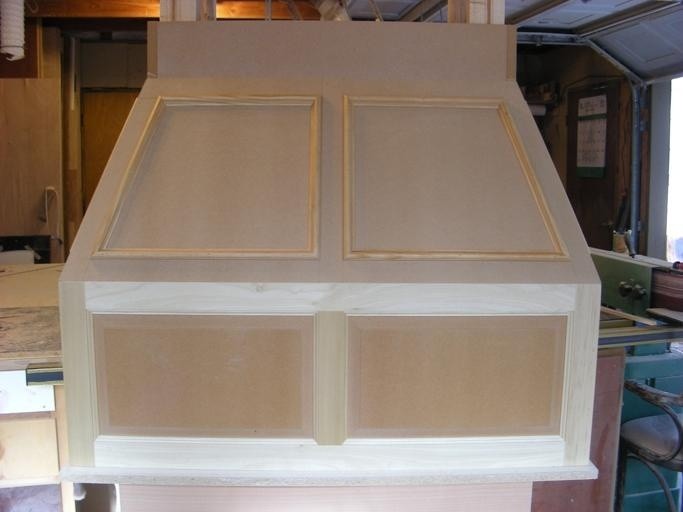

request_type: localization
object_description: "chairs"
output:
[616,380,683,512]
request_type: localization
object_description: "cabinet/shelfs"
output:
[618,350,683,511]
[532,346,625,510]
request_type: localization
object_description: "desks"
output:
[0,263,77,512]
